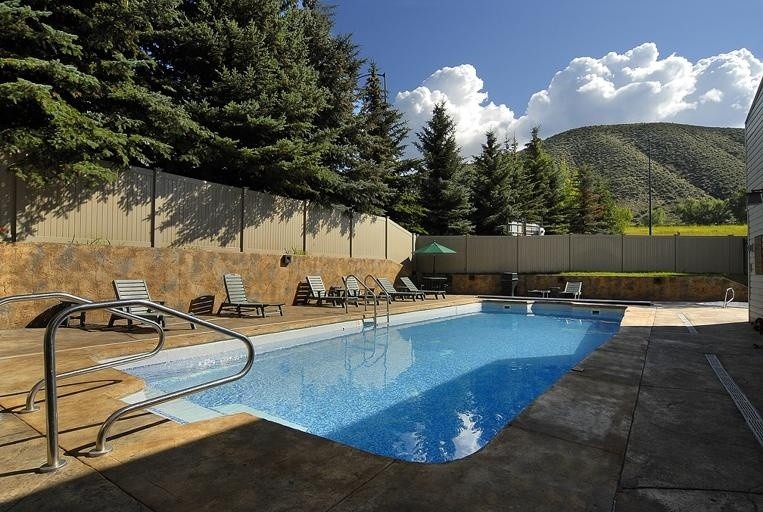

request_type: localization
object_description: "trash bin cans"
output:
[500,272,518,296]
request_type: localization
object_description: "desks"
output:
[421,276,447,291]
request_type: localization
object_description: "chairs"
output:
[341,276,392,304]
[399,276,446,299]
[216,273,285,318]
[107,279,198,333]
[304,275,358,307]
[376,278,424,302]
[526,286,560,298]
[558,281,583,298]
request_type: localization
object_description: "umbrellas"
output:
[411,239,458,277]
